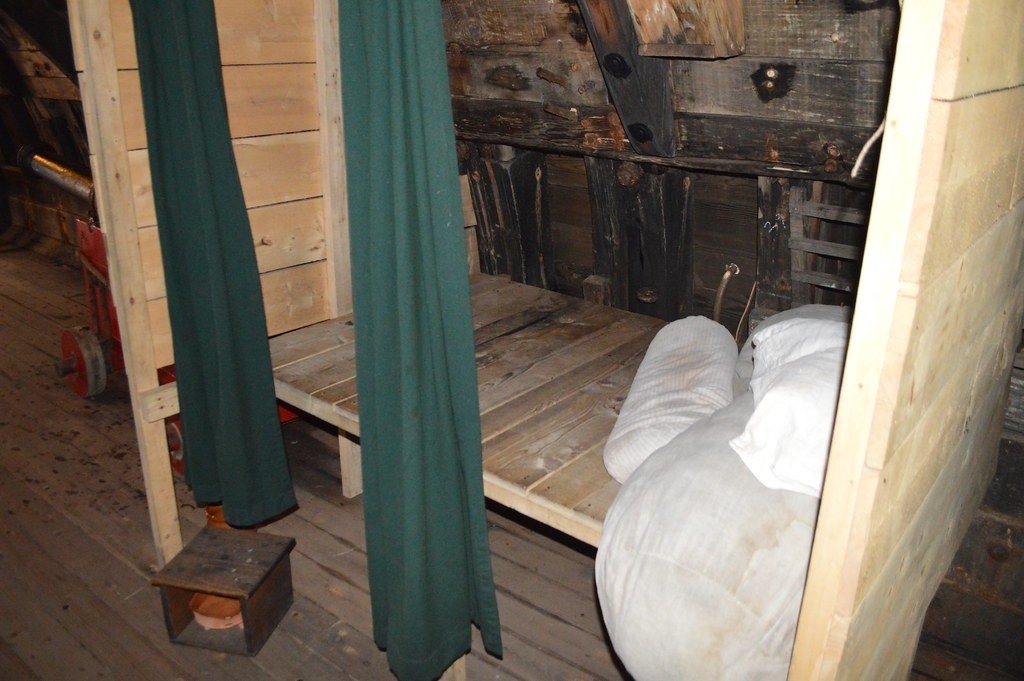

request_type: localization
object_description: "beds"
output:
[262,271,747,555]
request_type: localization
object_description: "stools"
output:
[147,525,297,658]
